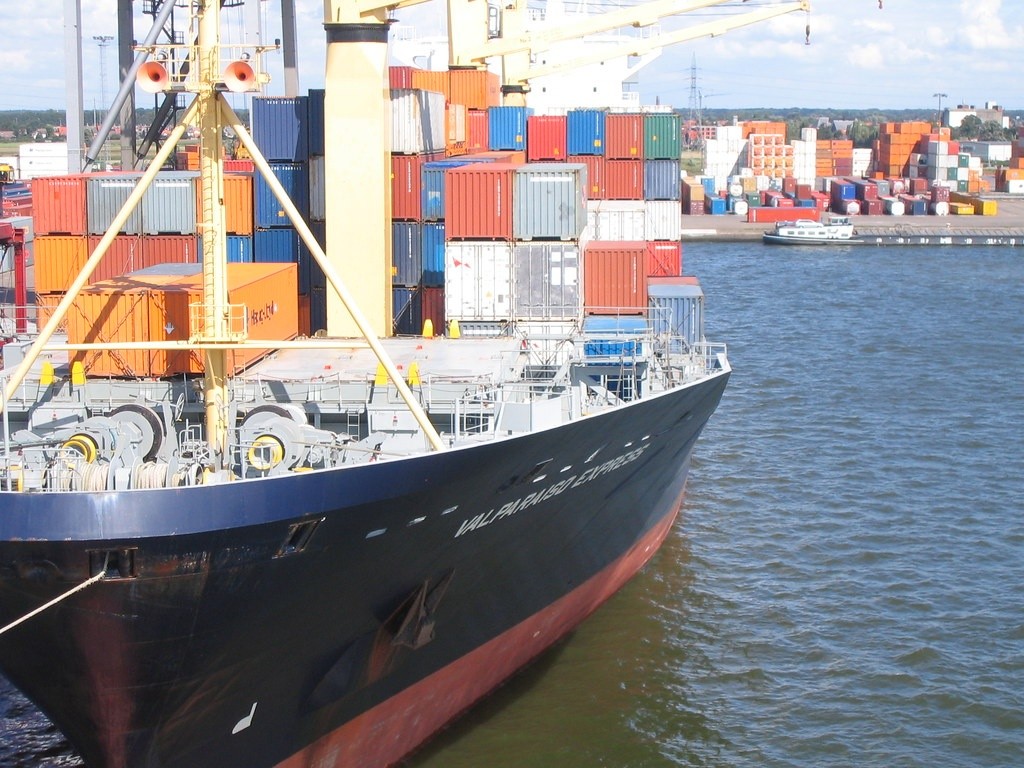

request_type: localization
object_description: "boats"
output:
[0,0,731,768]
[763,222,864,245]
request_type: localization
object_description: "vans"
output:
[829,217,851,226]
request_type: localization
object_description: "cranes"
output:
[325,0,883,340]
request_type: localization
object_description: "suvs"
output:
[796,219,824,228]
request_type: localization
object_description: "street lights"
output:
[933,92,948,187]
